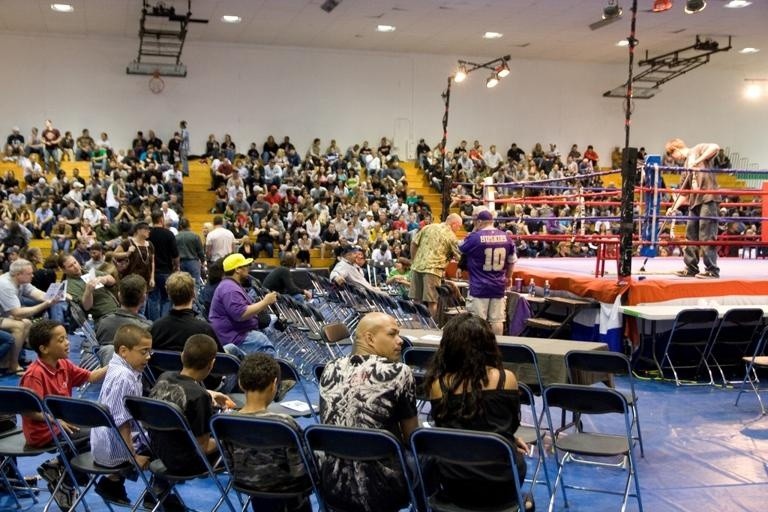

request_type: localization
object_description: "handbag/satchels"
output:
[111,257,129,273]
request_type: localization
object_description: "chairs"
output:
[735,326,768,415]
[657,309,718,387]
[699,307,764,388]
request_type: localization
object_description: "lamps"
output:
[453,53,511,88]
[685,0,706,14]
[602,0,623,20]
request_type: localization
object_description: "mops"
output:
[639,171,691,280]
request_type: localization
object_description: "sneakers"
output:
[0,475,37,490]
[675,270,695,277]
[144,491,189,512]
[37,459,76,512]
[274,379,296,402]
[695,271,719,279]
[19,357,32,365]
[95,476,131,504]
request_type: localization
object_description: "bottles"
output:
[544,280,550,298]
[529,278,535,297]
[217,400,231,413]
[455,268,464,281]
[87,312,95,330]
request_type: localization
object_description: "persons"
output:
[1,118,768,512]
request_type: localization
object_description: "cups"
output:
[516,278,523,293]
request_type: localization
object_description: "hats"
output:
[223,253,254,272]
[39,178,46,185]
[253,185,262,192]
[343,245,357,253]
[73,182,84,188]
[86,243,100,251]
[477,210,492,220]
[366,211,373,216]
[131,221,148,232]
[5,248,17,254]
